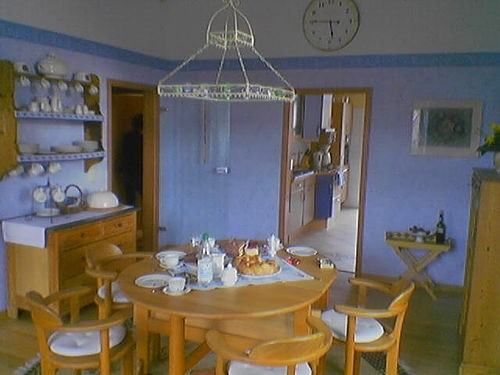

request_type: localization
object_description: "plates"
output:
[17,144,40,154]
[162,287,191,296]
[74,72,91,82]
[51,145,82,153]
[72,141,98,152]
[159,263,182,269]
[135,274,171,288]
[156,251,187,260]
[286,247,317,256]
[37,208,60,217]
[236,262,282,278]
[14,62,31,73]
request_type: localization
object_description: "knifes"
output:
[300,148,312,167]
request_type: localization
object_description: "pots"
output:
[34,51,74,79]
[35,96,49,108]
[341,95,350,103]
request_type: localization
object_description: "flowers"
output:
[475,123,500,157]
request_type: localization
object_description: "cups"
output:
[32,102,40,112]
[75,82,84,92]
[51,186,65,202]
[58,80,68,90]
[169,278,186,292]
[33,188,47,202]
[83,105,88,114]
[219,261,239,287]
[75,104,82,113]
[208,240,214,246]
[29,163,45,175]
[20,77,30,87]
[159,255,179,267]
[41,79,50,89]
[47,162,62,174]
[89,85,98,95]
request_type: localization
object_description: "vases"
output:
[494,152,500,175]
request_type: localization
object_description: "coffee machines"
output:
[323,147,333,170]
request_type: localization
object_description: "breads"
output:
[232,255,278,276]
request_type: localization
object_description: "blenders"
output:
[313,152,322,173]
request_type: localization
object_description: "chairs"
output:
[190,316,334,375]
[316,278,415,375]
[84,241,154,320]
[25,289,134,375]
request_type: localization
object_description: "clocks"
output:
[303,0,360,51]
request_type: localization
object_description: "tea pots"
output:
[56,184,82,213]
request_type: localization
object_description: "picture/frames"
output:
[410,99,482,157]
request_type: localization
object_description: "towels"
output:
[167,255,314,291]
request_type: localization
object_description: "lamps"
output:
[156,0,297,103]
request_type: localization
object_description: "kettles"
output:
[313,152,322,169]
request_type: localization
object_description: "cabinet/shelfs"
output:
[290,174,317,235]
[456,169,500,375]
[1,209,140,319]
[0,60,106,182]
[315,175,342,218]
[330,103,353,203]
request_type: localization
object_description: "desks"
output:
[384,232,452,300]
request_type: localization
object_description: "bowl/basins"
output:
[87,190,120,208]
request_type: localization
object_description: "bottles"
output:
[436,210,445,242]
[50,92,62,112]
[198,233,213,288]
[205,251,226,280]
[191,233,196,248]
[196,239,200,246]
[41,100,51,112]
